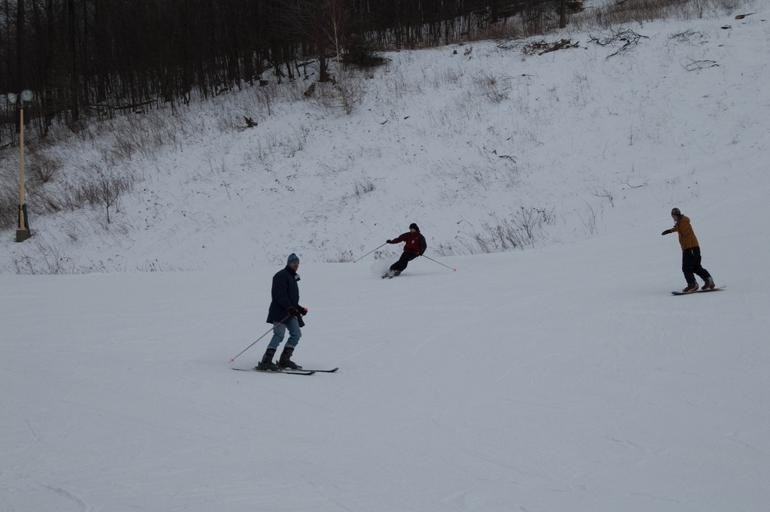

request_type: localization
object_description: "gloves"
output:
[416,251,421,256]
[662,230,671,235]
[387,240,392,243]
[286,305,307,316]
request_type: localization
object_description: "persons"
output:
[662,207,715,292]
[262,253,307,371]
[387,223,426,275]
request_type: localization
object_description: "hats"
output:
[671,208,680,213]
[410,223,418,228]
[287,253,299,261]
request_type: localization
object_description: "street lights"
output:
[6,85,34,242]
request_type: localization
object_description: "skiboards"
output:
[671,282,728,296]
[233,366,339,376]
[381,268,401,279]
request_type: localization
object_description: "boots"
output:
[382,269,400,279]
[257,346,303,371]
[702,275,715,290]
[683,277,699,292]
